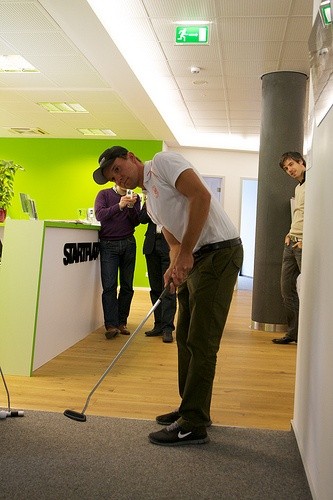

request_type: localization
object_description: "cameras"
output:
[127,189,132,199]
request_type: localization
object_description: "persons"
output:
[140,200,177,342]
[272,151,306,344]
[94,186,141,338]
[93,146,244,446]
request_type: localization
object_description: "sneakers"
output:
[272,333,297,344]
[155,409,213,427]
[148,416,210,446]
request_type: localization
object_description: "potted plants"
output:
[0,159,23,223]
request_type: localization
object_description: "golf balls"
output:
[0,410,6,419]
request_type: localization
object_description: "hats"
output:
[93,145,128,185]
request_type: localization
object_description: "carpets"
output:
[0,407,314,500]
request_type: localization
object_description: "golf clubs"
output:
[63,279,174,422]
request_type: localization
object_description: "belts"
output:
[193,237,241,253]
[288,235,303,242]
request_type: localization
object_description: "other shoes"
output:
[145,327,163,336]
[117,323,130,335]
[105,325,121,339]
[162,327,173,342]
